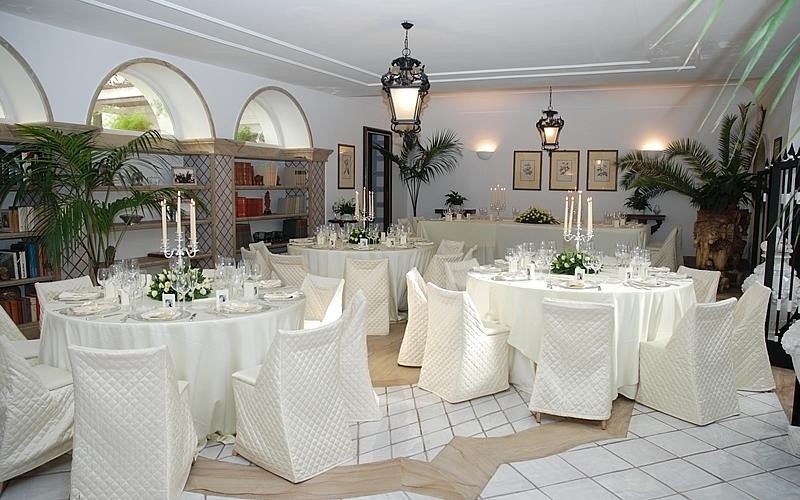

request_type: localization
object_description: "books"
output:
[235,162,308,250]
[1,142,56,324]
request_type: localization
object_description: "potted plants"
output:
[444,190,469,209]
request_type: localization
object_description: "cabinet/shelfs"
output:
[0,137,61,339]
[234,155,311,258]
[90,148,214,277]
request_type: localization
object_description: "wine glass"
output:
[168,256,199,308]
[441,184,521,222]
[615,243,651,283]
[214,255,258,299]
[504,239,557,283]
[602,207,629,229]
[97,257,147,312]
[654,204,661,216]
[316,218,415,249]
[583,249,603,283]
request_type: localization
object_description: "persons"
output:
[174,171,194,183]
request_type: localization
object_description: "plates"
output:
[496,272,531,282]
[141,309,191,321]
[560,281,598,289]
[216,301,272,315]
[51,288,121,318]
[633,275,665,287]
[265,291,300,303]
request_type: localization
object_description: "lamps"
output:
[382,21,430,138]
[536,86,565,152]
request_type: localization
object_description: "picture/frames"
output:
[548,150,580,192]
[338,144,356,189]
[171,166,197,186]
[587,150,618,191]
[513,150,542,191]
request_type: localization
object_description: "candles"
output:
[161,201,197,244]
[356,190,374,218]
[563,194,592,236]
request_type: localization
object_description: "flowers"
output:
[331,196,358,215]
[515,205,559,224]
[347,226,377,244]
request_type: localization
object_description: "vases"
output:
[340,213,353,221]
